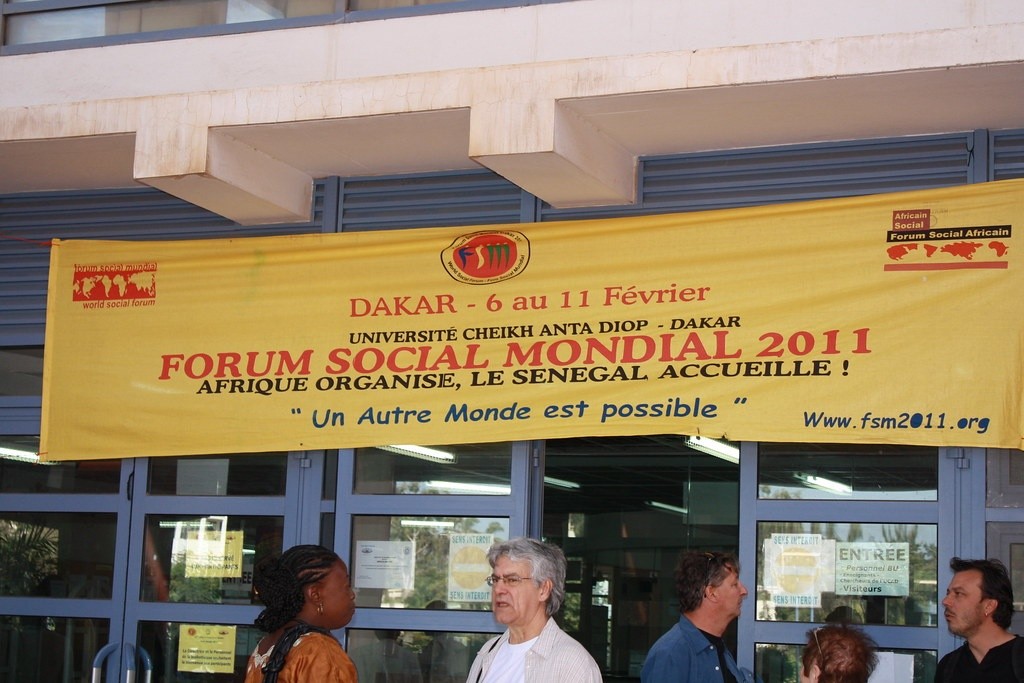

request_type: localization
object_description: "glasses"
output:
[813,627,825,670]
[485,575,533,587]
[703,552,716,599]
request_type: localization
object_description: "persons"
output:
[933,556,1024,683]
[638,548,749,683]
[350,598,469,683]
[799,627,879,683]
[244,542,359,683]
[755,646,793,683]
[860,592,937,683]
[466,537,603,683]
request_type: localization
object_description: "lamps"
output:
[795,471,851,497]
[0,440,61,467]
[683,435,741,465]
[377,444,459,466]
